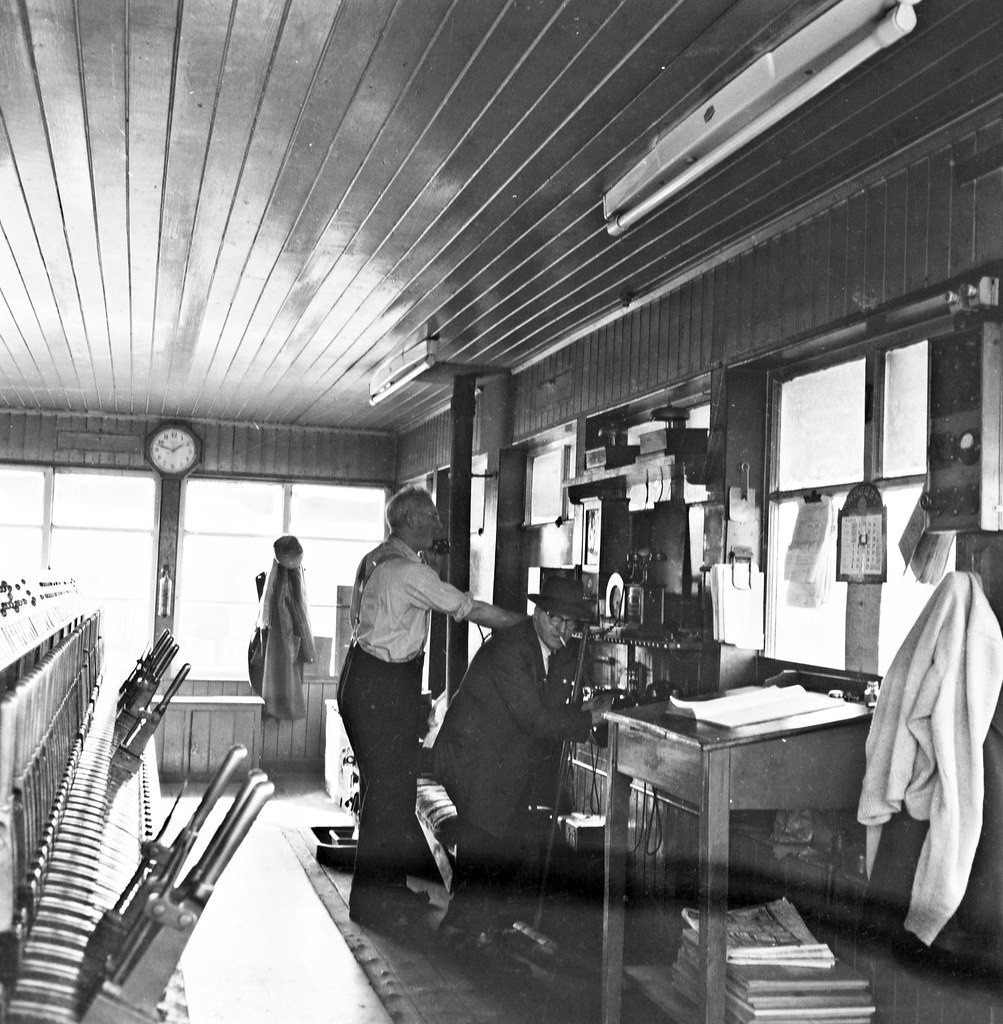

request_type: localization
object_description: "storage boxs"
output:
[584,446,631,468]
[638,427,709,454]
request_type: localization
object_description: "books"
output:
[672,897,877,1024]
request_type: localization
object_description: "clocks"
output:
[143,424,202,480]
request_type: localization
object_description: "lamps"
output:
[369,339,439,406]
[603,0,921,237]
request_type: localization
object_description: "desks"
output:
[600,689,873,1024]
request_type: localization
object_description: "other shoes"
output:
[432,930,533,985]
[349,884,430,929]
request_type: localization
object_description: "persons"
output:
[431,576,614,972]
[336,490,529,924]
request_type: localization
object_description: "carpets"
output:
[280,824,1003,1024]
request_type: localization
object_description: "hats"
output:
[526,576,597,611]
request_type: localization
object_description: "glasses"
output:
[544,608,579,631]
[415,511,440,522]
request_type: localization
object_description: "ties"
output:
[547,651,556,681]
